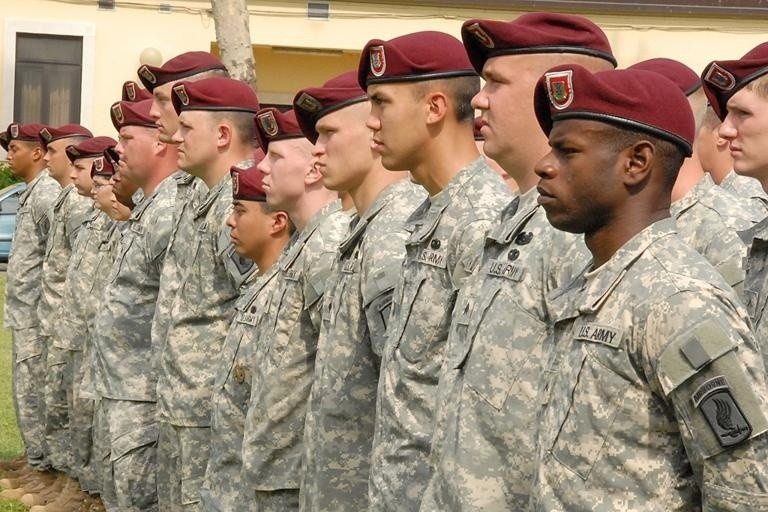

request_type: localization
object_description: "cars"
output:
[1,182,23,259]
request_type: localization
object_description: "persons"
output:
[0,7,767,512]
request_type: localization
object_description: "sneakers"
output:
[0,453,104,511]
